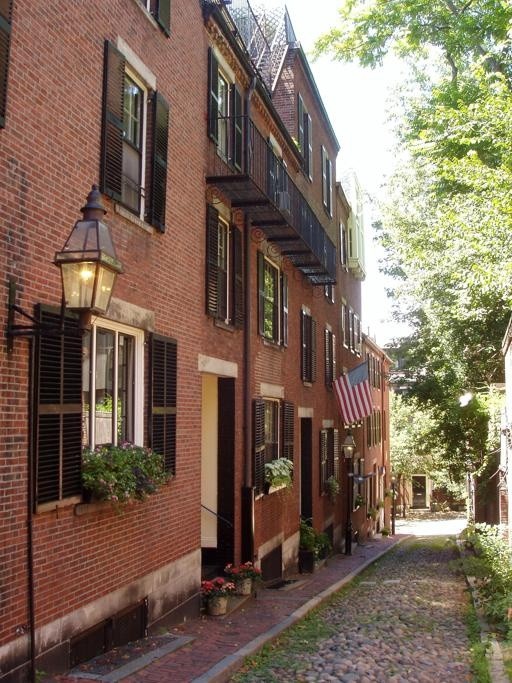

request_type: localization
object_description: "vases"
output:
[237,578,252,596]
[208,597,227,615]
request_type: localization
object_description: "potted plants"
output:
[381,528,391,541]
[300,517,331,573]
[264,457,295,495]
[326,475,341,497]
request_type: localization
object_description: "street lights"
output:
[340,429,357,554]
[390,470,397,534]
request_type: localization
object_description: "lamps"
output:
[8,184,124,355]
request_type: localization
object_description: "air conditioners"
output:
[275,190,291,215]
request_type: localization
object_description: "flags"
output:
[330,359,378,427]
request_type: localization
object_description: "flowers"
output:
[81,441,168,510]
[224,561,264,587]
[202,575,235,599]
